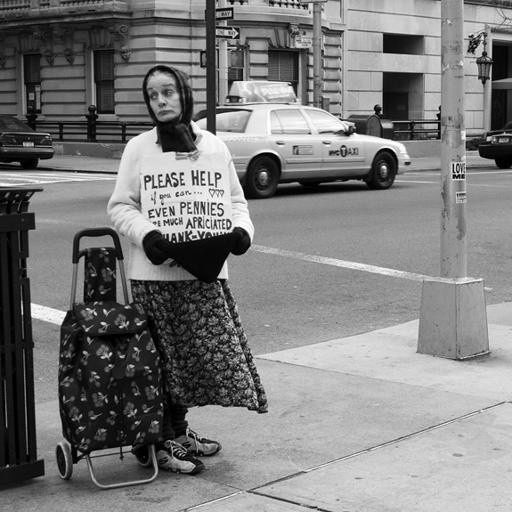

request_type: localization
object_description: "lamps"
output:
[470,31,493,87]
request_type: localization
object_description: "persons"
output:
[106,66,254,476]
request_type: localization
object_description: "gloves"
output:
[142,230,170,265]
[230,227,251,256]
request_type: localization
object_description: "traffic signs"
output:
[205,8,234,20]
[215,27,241,40]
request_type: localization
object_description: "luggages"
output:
[55,226,164,489]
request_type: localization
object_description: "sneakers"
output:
[135,426,222,476]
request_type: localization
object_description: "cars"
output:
[478,122,511,170]
[0,116,54,169]
[191,102,412,198]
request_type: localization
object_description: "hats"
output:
[169,232,233,285]
